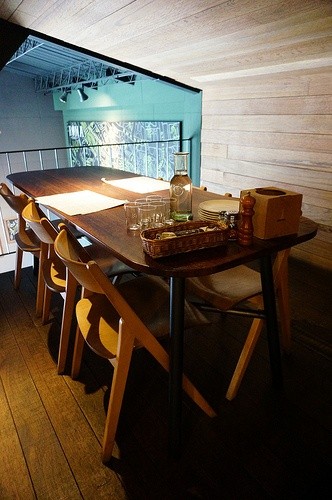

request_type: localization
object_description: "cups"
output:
[160,198,176,225]
[135,199,151,225]
[146,195,162,219]
[148,201,165,227]
[124,202,140,230]
[138,205,154,233]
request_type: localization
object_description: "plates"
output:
[198,200,239,222]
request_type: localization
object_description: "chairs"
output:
[0,182,292,466]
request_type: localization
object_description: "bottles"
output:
[170,152,193,221]
[218,211,237,240]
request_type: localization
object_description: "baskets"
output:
[140,220,228,258]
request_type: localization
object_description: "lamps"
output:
[59,91,68,103]
[76,88,88,102]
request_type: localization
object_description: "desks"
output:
[6,166,320,490]
